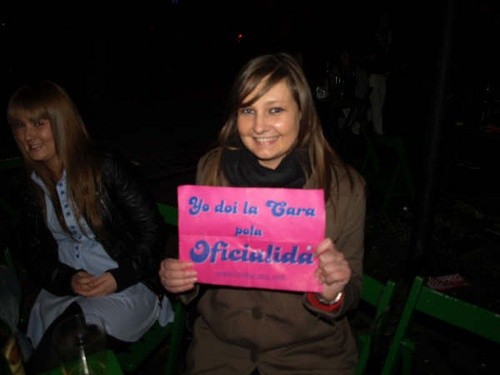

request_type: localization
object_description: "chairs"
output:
[354,273,397,375]
[356,118,414,208]
[381,273,500,375]
[106,201,185,375]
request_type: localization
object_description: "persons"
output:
[158,53,364,375]
[350,13,396,136]
[0,81,174,375]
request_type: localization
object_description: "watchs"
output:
[317,292,343,306]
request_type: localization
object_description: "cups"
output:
[54,313,109,375]
